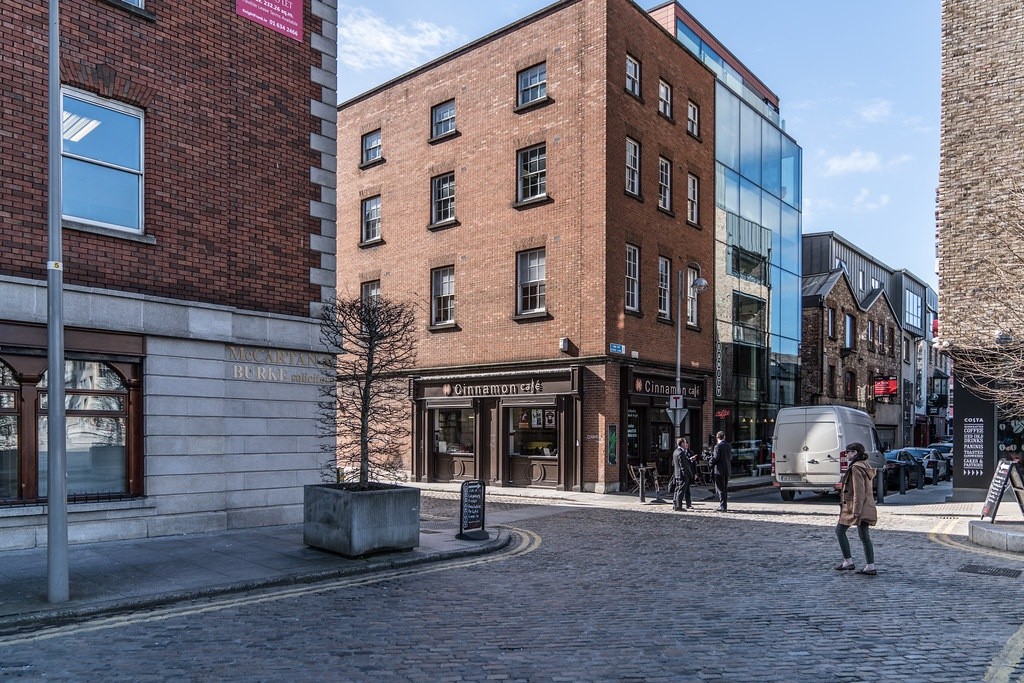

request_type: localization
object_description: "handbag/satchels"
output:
[667,473,676,493]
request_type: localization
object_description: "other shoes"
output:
[854,567,876,575]
[686,505,694,509]
[715,508,726,512]
[833,563,855,570]
[673,507,687,511]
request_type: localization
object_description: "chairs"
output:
[627,464,651,494]
[699,460,713,486]
[646,462,669,492]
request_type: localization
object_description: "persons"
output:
[757,441,769,464]
[672,437,697,511]
[709,431,731,511]
[836,443,877,575]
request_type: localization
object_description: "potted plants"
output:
[752,463,758,477]
[299,294,420,558]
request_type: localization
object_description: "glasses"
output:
[846,450,854,453]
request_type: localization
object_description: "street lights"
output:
[674,262,708,453]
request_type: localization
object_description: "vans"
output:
[770,405,888,501]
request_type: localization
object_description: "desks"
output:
[630,466,658,493]
[695,464,709,486]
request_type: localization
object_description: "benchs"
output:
[757,463,772,477]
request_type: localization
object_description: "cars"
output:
[906,446,949,484]
[927,443,955,476]
[728,439,765,465]
[883,449,926,491]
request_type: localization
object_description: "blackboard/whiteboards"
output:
[982,459,1024,517]
